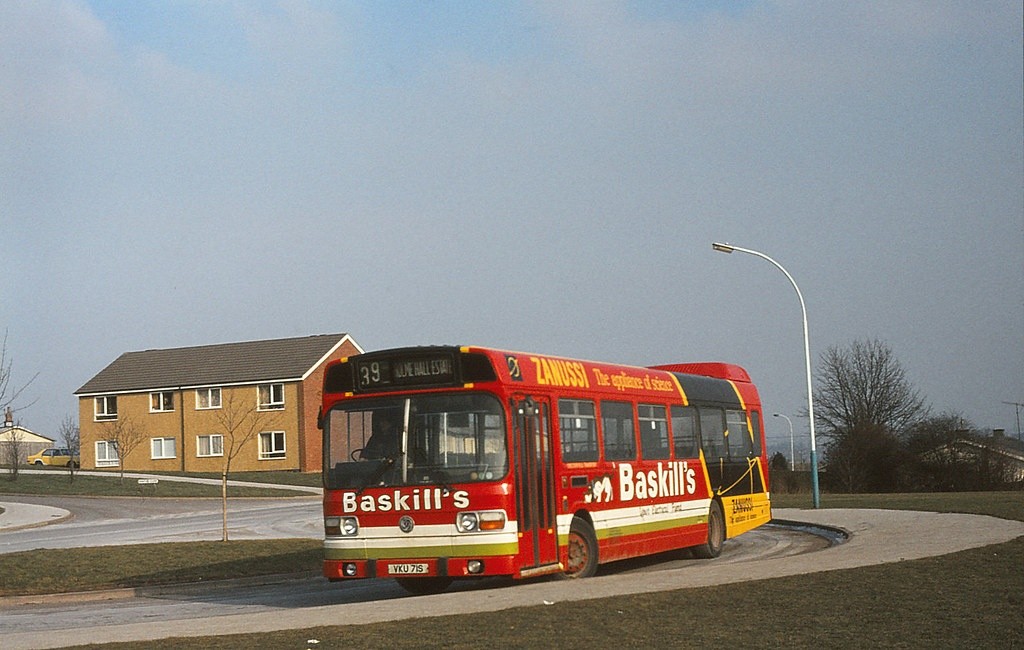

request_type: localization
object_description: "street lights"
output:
[772,412,795,471]
[711,241,820,509]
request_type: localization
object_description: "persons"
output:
[359,412,402,484]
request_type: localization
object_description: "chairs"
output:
[441,445,747,467]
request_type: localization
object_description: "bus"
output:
[315,344,773,594]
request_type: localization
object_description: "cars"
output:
[27,446,80,469]
